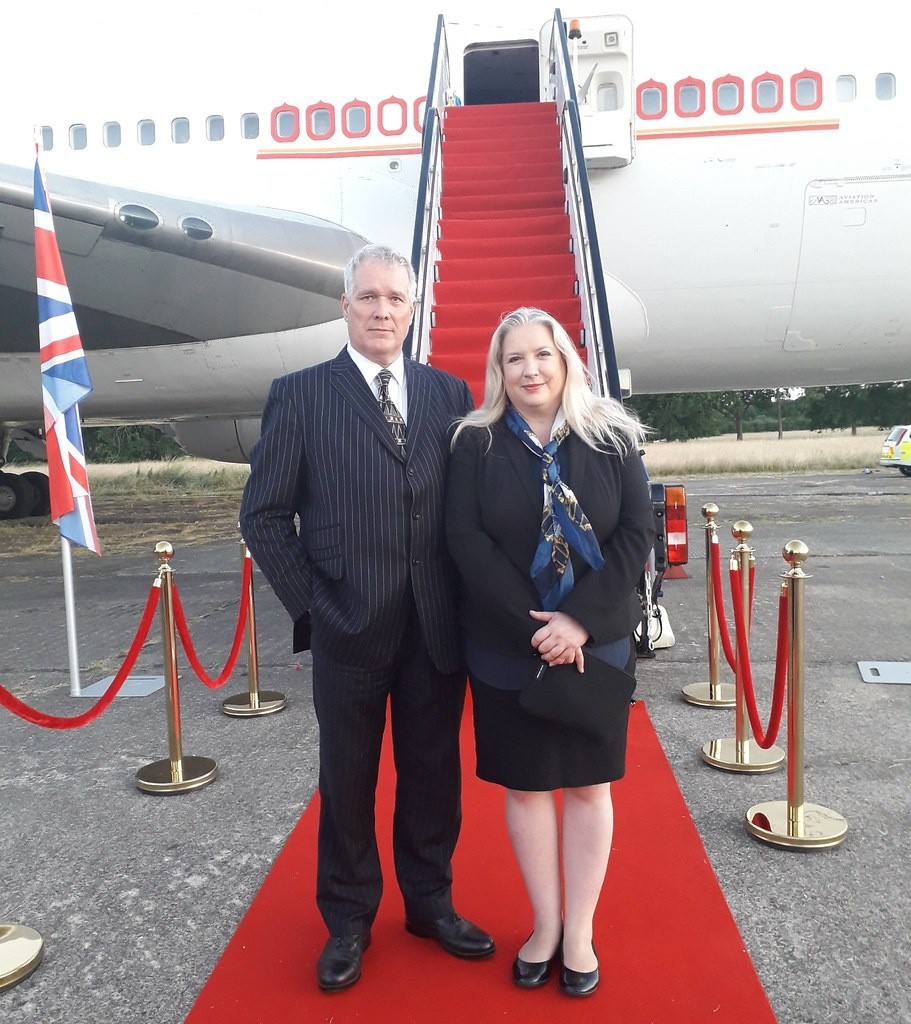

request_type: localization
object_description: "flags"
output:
[32,143,102,559]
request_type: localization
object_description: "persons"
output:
[442,305,653,998]
[238,244,499,992]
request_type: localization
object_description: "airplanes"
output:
[0,0,911,522]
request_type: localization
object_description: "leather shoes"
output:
[405,911,496,958]
[317,932,371,991]
[558,926,599,996]
[513,929,551,987]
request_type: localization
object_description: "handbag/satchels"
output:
[518,650,637,745]
[634,605,676,648]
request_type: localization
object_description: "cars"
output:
[879,425,910,476]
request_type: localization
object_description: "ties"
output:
[504,406,605,611]
[375,369,408,462]
